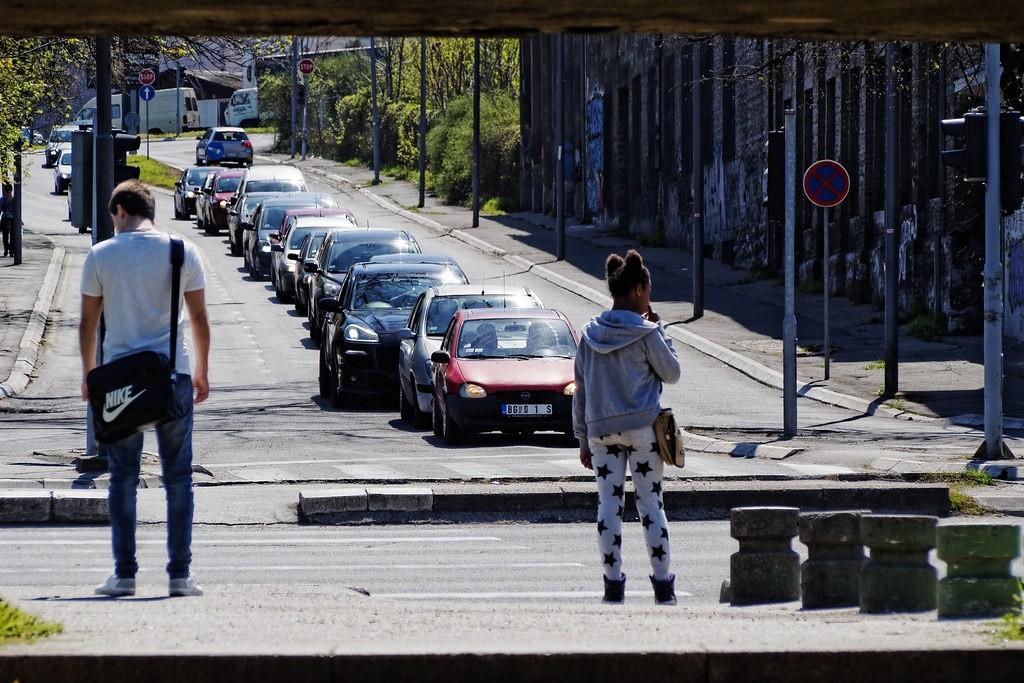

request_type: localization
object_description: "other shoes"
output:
[4,245,14,256]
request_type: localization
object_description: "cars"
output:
[304,230,423,346]
[240,199,328,282]
[397,285,545,430]
[227,164,338,255]
[268,209,395,316]
[203,170,244,234]
[21,125,92,195]
[195,127,253,167]
[174,166,228,221]
[369,253,460,268]
[318,260,471,409]
[194,171,215,229]
[431,307,580,446]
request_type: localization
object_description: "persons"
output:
[0,184,13,257]
[572,250,681,606]
[477,324,502,355]
[79,181,210,596]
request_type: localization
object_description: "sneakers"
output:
[94,573,136,596]
[168,571,204,596]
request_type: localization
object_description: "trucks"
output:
[224,88,280,128]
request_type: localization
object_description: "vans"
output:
[71,88,201,135]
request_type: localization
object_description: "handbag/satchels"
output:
[652,407,685,469]
[86,350,186,445]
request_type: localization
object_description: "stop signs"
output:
[139,68,155,85]
[299,59,315,75]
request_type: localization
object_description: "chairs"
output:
[526,322,557,351]
[436,300,459,323]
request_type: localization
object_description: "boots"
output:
[601,572,627,604]
[648,573,677,605]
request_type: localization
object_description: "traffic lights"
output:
[1001,111,1024,210]
[112,129,142,188]
[940,113,987,181]
